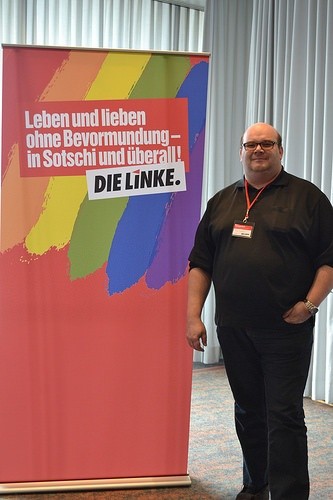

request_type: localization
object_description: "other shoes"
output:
[235,486,270,500]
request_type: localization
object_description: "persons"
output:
[185,123,333,500]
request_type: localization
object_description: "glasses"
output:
[241,140,279,150]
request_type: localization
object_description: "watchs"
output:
[302,299,318,315]
